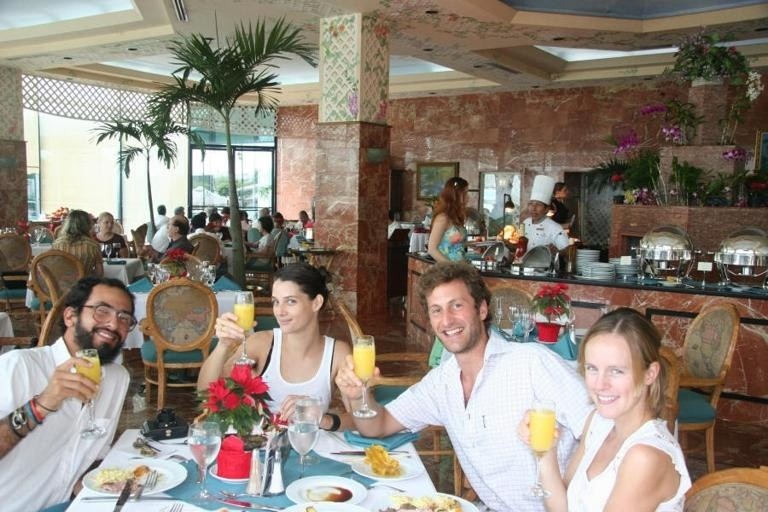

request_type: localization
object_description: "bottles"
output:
[565,322,576,346]
[270,446,285,495]
[245,447,262,497]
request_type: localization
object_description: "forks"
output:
[169,502,185,512]
[130,467,161,502]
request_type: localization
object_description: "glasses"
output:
[453,179,466,189]
[77,303,136,332]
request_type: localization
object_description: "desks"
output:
[62,429,437,511]
[406,250,768,428]
[453,325,574,465]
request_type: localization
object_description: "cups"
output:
[294,393,324,426]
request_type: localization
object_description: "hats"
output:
[530,175,556,205]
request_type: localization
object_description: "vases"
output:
[216,431,259,480]
[690,70,724,85]
[535,322,559,341]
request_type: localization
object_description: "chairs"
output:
[655,300,741,472]
[1,214,341,412]
[338,297,442,464]
[685,465,768,512]
[489,287,536,328]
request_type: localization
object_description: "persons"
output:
[29,204,314,311]
[196,262,378,431]
[335,261,597,512]
[519,175,569,265]
[548,182,571,235]
[0,276,137,512]
[518,307,694,512]
[427,176,475,370]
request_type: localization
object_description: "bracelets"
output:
[324,412,341,431]
[9,396,57,440]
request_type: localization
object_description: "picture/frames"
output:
[415,160,460,202]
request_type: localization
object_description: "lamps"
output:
[501,193,515,243]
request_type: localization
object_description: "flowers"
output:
[720,71,764,145]
[722,149,755,196]
[633,96,706,146]
[612,133,659,188]
[537,284,569,318]
[204,365,272,412]
[672,26,753,82]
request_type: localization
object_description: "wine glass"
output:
[74,347,107,440]
[99,242,123,263]
[1,227,48,246]
[288,414,319,475]
[233,290,258,367]
[145,257,219,290]
[353,334,379,419]
[525,396,558,503]
[492,294,537,346]
[189,421,222,500]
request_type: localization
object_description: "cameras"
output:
[140,408,188,441]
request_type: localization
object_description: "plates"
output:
[208,462,250,486]
[281,501,366,511]
[353,457,424,481]
[574,248,639,279]
[533,338,557,345]
[284,475,368,506]
[102,500,207,512]
[82,458,190,497]
[371,490,479,512]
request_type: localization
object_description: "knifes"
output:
[327,449,408,456]
[112,475,134,510]
[205,494,284,511]
